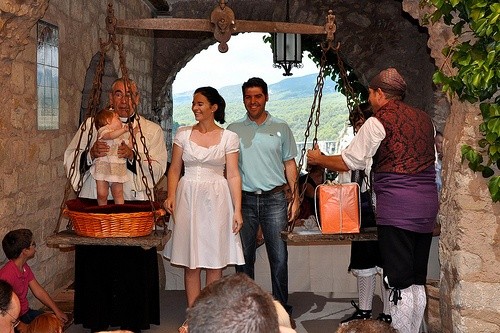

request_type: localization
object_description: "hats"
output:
[368,67,407,95]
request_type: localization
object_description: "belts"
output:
[242,184,283,196]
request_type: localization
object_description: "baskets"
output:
[65,198,168,238]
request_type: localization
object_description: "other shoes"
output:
[340,307,374,323]
[291,317,296,329]
[178,320,189,333]
[376,313,393,325]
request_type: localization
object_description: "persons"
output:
[89,109,134,206]
[308,68,442,333]
[0,279,21,333]
[186,273,281,333]
[226,77,300,329]
[27,313,64,333]
[63,78,168,333]
[162,86,246,333]
[298,151,328,219]
[284,183,311,226]
[435,130,443,154]
[340,102,393,325]
[0,228,68,324]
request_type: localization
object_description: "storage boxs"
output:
[318,183,361,235]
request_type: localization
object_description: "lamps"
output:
[272,0,302,77]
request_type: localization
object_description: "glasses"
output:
[0,306,21,327]
[31,241,36,248]
